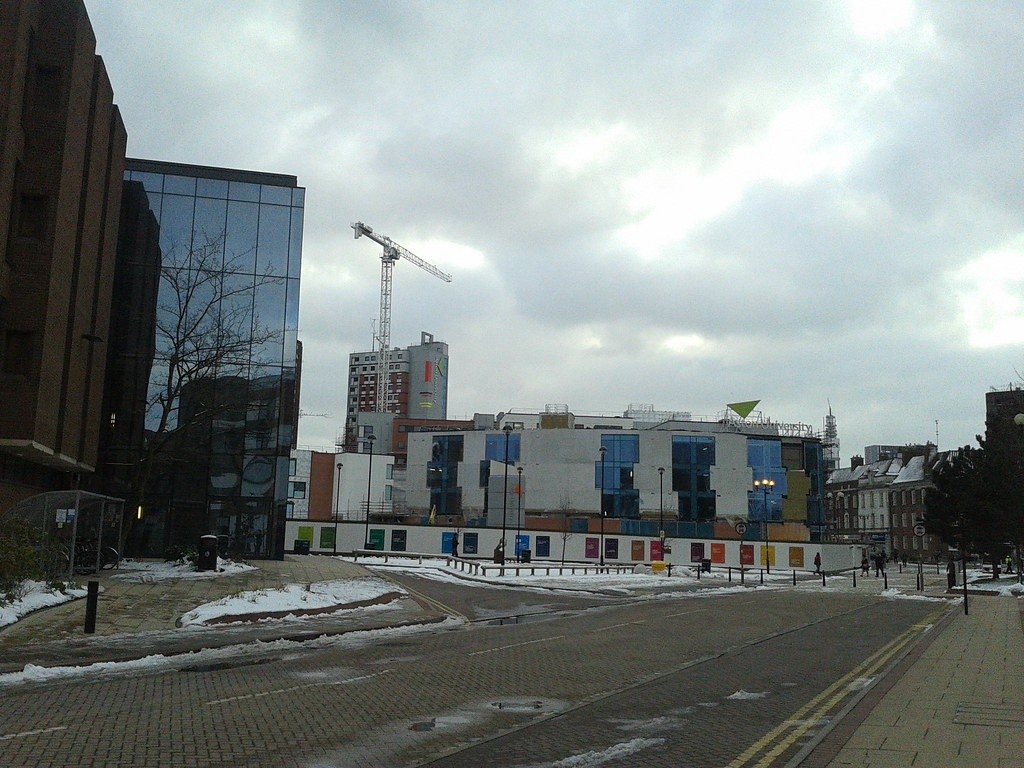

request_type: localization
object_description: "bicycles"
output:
[47,533,120,577]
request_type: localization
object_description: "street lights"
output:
[657,466,666,561]
[364,435,377,557]
[827,492,845,543]
[333,463,344,556]
[753,479,775,574]
[501,426,513,565]
[517,466,524,563]
[599,446,608,573]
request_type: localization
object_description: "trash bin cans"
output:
[652,560,666,574]
[701,558,712,574]
[198,535,218,570]
[293,539,310,555]
[521,549,531,563]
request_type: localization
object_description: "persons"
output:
[901,551,909,567]
[946,555,956,589]
[450,533,459,561]
[860,557,870,577]
[813,552,821,576]
[875,549,887,577]
[1003,555,1014,574]
[891,549,899,564]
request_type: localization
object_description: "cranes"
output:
[351,219,452,413]
